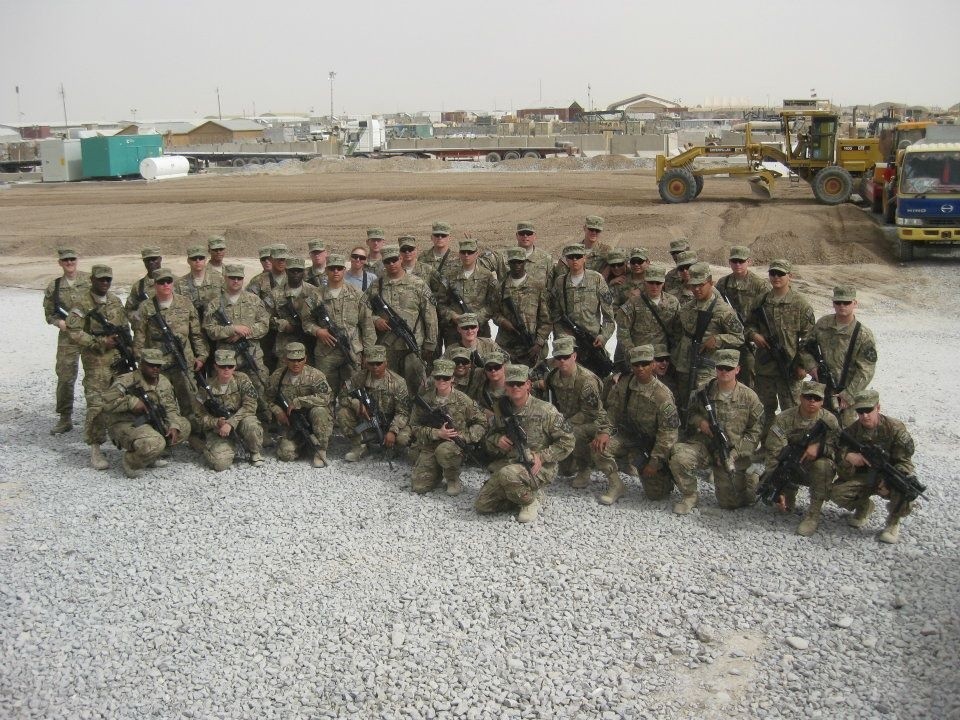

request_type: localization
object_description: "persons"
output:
[476,352,509,472]
[672,263,745,482]
[591,344,682,505]
[193,350,264,470]
[132,267,205,450]
[668,350,766,515]
[743,258,816,462]
[264,341,333,468]
[545,337,608,488]
[335,345,410,462]
[421,347,482,402]
[758,382,842,536]
[830,388,915,544]
[102,348,192,478]
[43,250,92,435]
[409,359,487,495]
[66,265,132,469]
[124,216,770,437]
[476,365,576,521]
[800,283,877,425]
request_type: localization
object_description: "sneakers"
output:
[151,458,168,468]
[251,451,264,467]
[90,444,109,470]
[122,452,144,478]
[313,449,326,468]
[345,444,369,461]
[49,413,72,435]
[436,459,901,544]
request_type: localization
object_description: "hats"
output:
[854,390,879,408]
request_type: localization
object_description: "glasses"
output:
[62,215,853,397]
[632,361,651,367]
[555,355,571,361]
[434,376,451,381]
[856,407,874,415]
[368,361,383,366]
[219,365,234,369]
[289,359,305,362]
[508,381,525,387]
[462,325,477,330]
[802,394,822,401]
[453,358,469,365]
[716,365,735,371]
[656,357,671,362]
[146,364,161,368]
[485,364,502,371]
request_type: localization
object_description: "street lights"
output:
[328,71,336,124]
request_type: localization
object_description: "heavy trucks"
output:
[342,120,580,162]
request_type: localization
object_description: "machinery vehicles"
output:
[658,108,937,205]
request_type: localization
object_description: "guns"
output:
[749,303,794,388]
[281,296,307,345]
[407,391,482,469]
[694,390,738,488]
[374,293,430,371]
[133,290,145,307]
[48,294,72,318]
[348,386,395,466]
[562,309,617,369]
[677,309,713,401]
[840,428,931,503]
[314,302,358,370]
[92,307,272,457]
[804,341,843,410]
[448,287,476,329]
[272,388,328,468]
[501,294,546,368]
[494,394,543,492]
[755,417,832,506]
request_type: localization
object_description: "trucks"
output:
[864,142,960,262]
